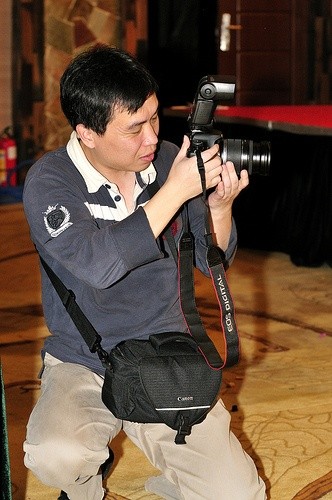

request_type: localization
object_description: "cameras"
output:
[185,75,273,178]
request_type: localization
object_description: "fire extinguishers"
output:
[0,126,16,187]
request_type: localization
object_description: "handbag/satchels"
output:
[101,331,223,445]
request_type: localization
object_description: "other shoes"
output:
[57,445,114,500]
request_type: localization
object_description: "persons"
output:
[22,44,269,500]
